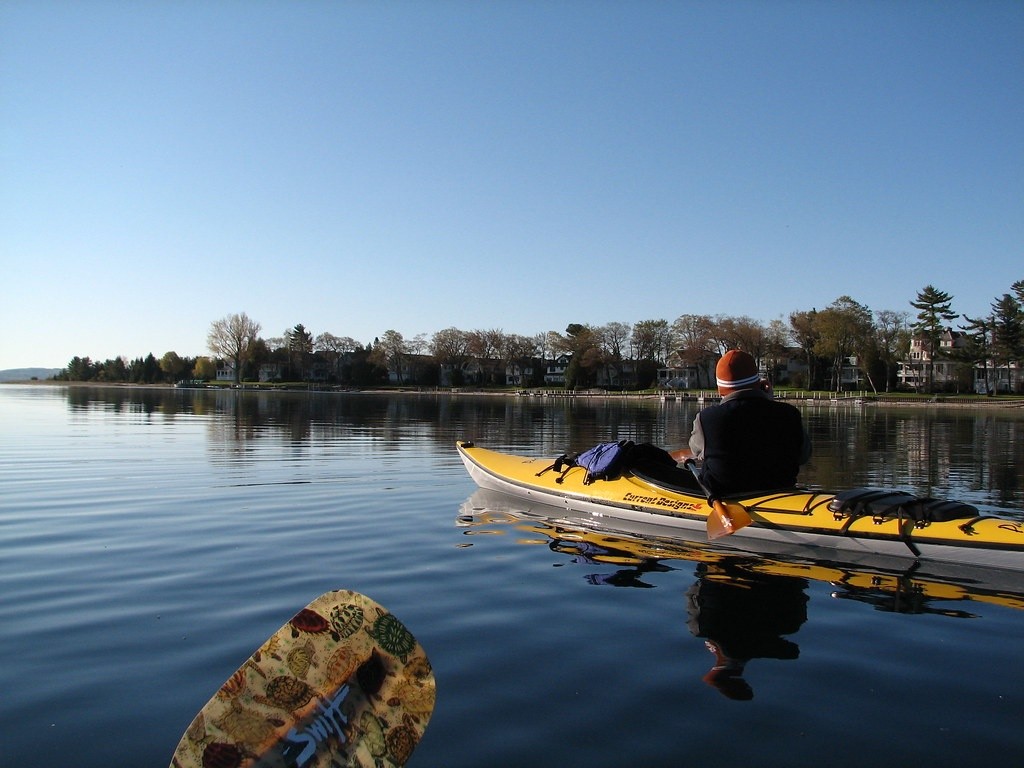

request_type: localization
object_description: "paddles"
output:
[671,445,756,543]
[158,585,438,767]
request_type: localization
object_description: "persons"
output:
[685,565,811,698]
[690,349,809,498]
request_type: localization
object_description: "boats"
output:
[455,440,1024,574]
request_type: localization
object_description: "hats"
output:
[715,350,760,396]
[705,659,755,700]
